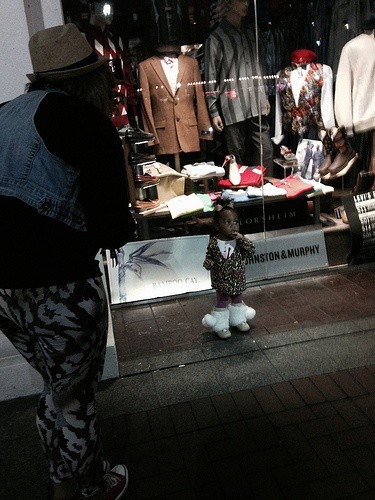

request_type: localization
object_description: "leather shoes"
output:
[310,212,337,226]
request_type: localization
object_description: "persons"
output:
[0,23,136,500]
[333,28,375,136]
[78,0,143,128]
[202,201,256,339]
[204,0,273,177]
[271,50,335,145]
[138,46,213,156]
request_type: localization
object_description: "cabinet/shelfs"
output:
[106,132,351,310]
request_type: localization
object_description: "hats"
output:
[26,23,109,82]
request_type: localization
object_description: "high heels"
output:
[221,154,241,186]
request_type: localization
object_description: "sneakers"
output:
[125,128,155,144]
[136,173,161,187]
[129,152,157,165]
[102,460,129,500]
[135,198,161,212]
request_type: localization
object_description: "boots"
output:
[318,126,359,180]
[202,300,257,339]
